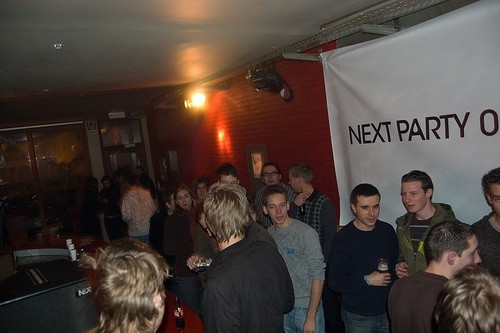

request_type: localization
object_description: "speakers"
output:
[246,69,279,91]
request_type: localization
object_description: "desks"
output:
[81,241,204,333]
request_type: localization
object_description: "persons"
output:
[324,183,399,333]
[435,265,500,333]
[85,237,172,333]
[395,170,456,279]
[469,167,500,276]
[262,186,326,333]
[388,221,482,333]
[77,161,337,317]
[204,183,295,333]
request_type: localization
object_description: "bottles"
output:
[174,296,185,330]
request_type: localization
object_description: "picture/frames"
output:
[245,144,270,182]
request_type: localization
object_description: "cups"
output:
[377,258,388,273]
[398,255,407,265]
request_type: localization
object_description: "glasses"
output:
[262,170,279,177]
